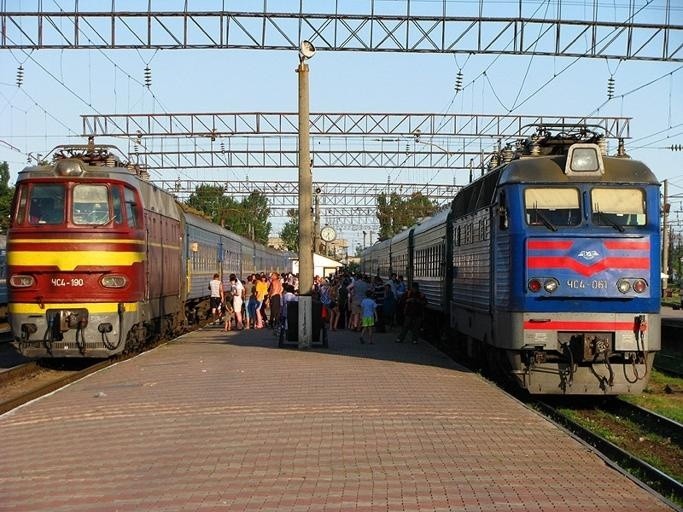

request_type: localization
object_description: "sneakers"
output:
[395,338,420,344]
[359,336,376,345]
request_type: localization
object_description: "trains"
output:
[4,143,297,362]
[360,123,662,396]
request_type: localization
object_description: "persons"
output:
[207,272,224,325]
[223,266,425,346]
